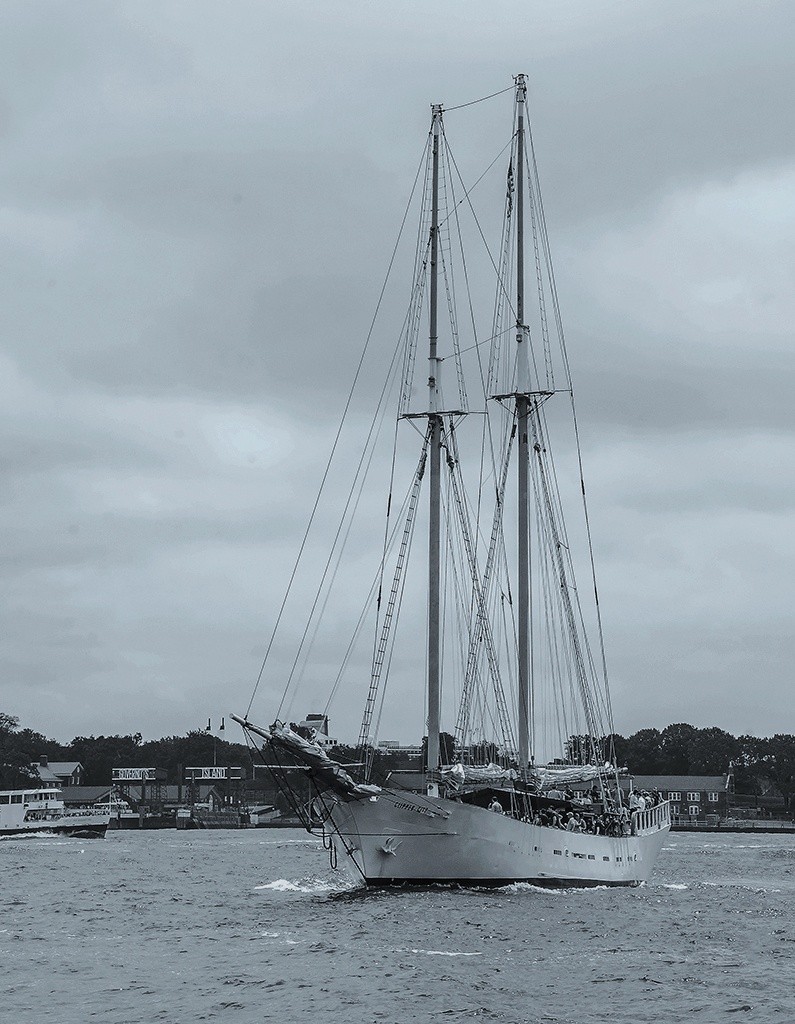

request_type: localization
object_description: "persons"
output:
[489,782,666,838]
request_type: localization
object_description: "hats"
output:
[566,812,573,815]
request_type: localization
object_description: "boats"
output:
[0,789,112,840]
[175,805,260,830]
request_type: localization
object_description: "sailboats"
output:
[228,69,677,887]
